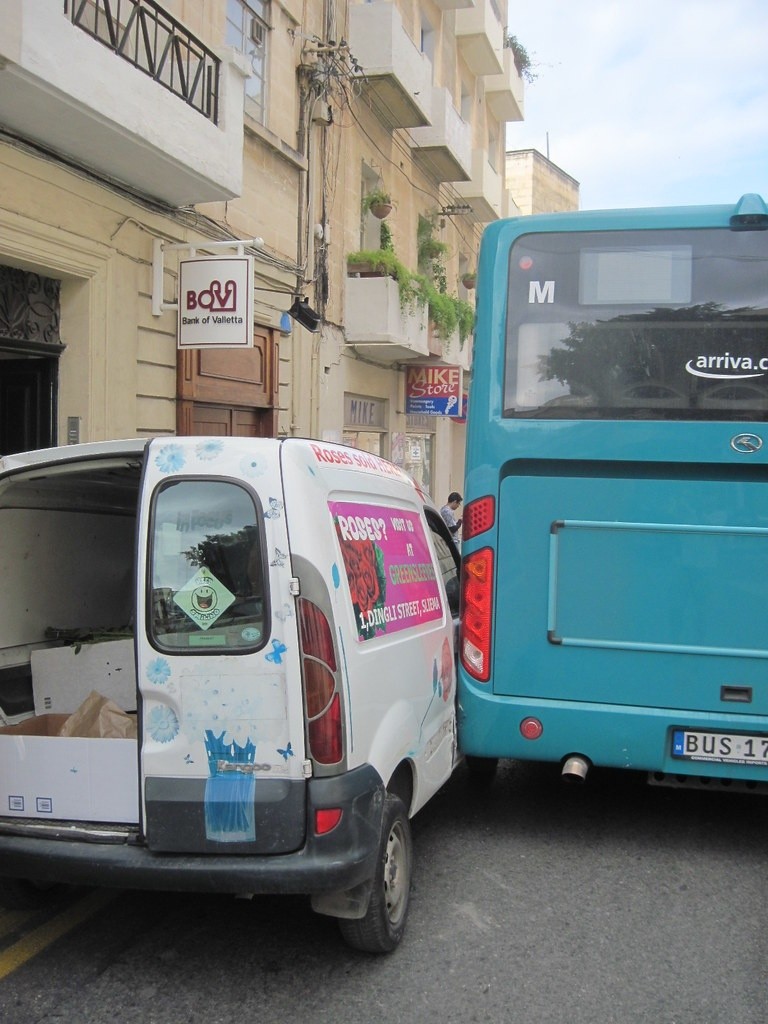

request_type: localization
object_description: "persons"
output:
[437,490,464,547]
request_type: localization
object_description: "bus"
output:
[453,190,768,799]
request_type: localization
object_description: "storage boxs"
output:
[0,713,140,827]
[30,636,138,718]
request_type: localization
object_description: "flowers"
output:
[420,636,453,739]
[332,513,385,640]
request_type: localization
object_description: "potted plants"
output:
[361,167,399,219]
[456,272,477,290]
[504,35,538,83]
[347,250,477,356]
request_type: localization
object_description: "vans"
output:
[0,435,500,953]
